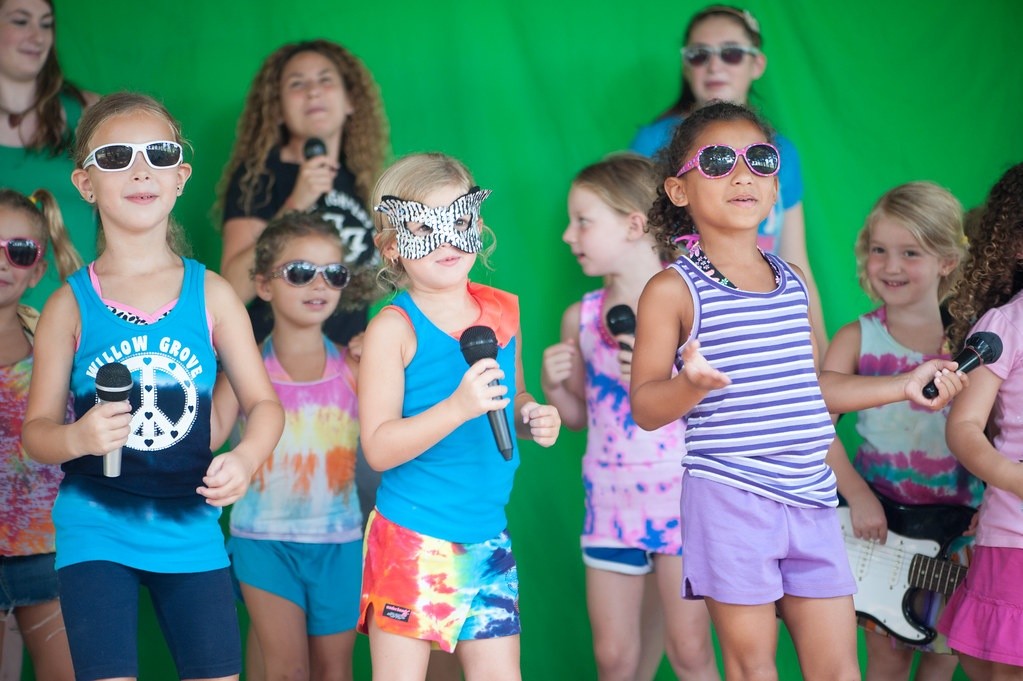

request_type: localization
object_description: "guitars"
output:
[775,485,981,649]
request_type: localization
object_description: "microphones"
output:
[460,325,513,460]
[304,137,329,208]
[922,332,1003,400]
[607,304,636,362]
[95,362,133,479]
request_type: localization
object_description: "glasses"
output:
[683,45,761,66]
[675,142,781,178]
[0,237,46,269]
[268,259,353,289]
[82,140,185,171]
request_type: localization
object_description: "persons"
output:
[208,208,368,681]
[631,95,969,681]
[632,3,829,681]
[21,92,286,681]
[357,153,563,681]
[0,187,83,681]
[818,161,1023,681]
[1,1,102,681]
[210,35,398,603]
[543,151,725,681]
